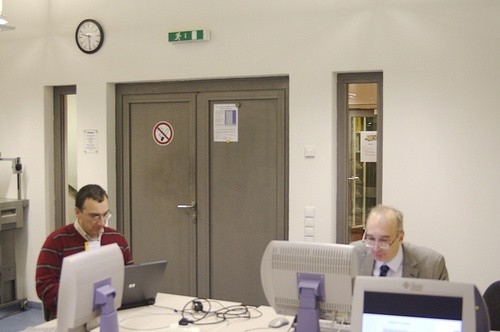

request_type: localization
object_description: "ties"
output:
[379,264,390,276]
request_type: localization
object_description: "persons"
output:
[349,205,449,281]
[36,185,134,321]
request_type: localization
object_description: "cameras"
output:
[55,242,124,332]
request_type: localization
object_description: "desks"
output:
[32,292,298,332]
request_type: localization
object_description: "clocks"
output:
[76,18,103,55]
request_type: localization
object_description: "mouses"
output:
[268,317,289,330]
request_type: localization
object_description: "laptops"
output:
[118,259,167,310]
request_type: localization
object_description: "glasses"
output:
[361,230,398,249]
[83,206,112,220]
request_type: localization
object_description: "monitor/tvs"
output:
[261,240,358,332]
[350,274,489,332]
